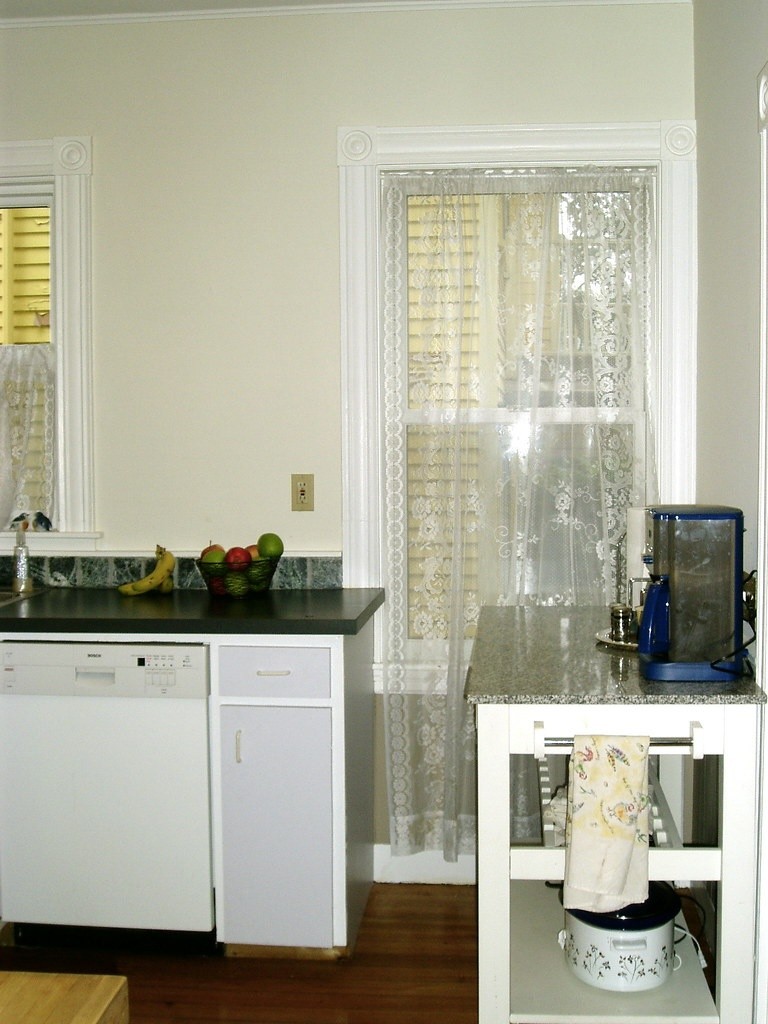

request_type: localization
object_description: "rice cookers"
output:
[545,881,707,992]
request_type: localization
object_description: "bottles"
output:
[608,602,632,642]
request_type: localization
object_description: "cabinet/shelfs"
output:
[463,604,768,1024]
[208,616,376,960]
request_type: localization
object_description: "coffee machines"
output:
[638,504,758,682]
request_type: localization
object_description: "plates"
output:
[594,628,638,651]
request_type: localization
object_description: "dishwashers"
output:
[0,642,214,950]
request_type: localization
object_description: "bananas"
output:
[118,544,175,596]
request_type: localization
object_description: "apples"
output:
[200,533,284,597]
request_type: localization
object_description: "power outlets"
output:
[291,474,314,512]
